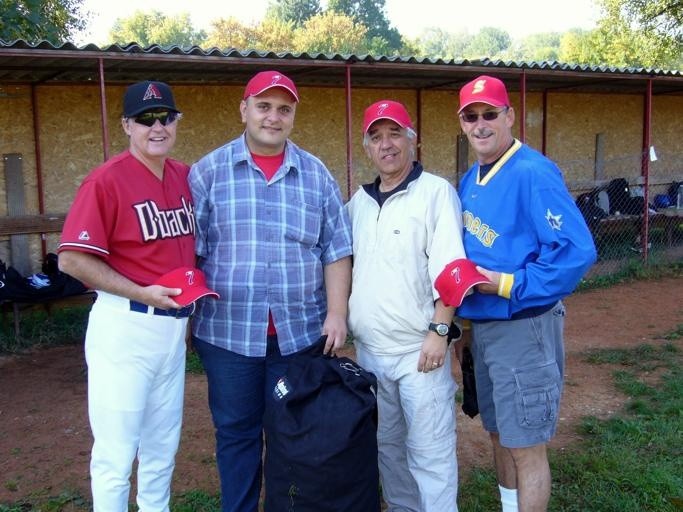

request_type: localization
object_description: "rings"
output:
[435,362,439,367]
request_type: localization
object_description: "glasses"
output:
[463,110,507,121]
[135,110,178,127]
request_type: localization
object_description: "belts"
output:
[128,297,199,319]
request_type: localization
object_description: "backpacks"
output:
[264,334,381,512]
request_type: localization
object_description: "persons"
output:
[187,70,353,511]
[454,74,597,512]
[342,97,470,512]
[56,81,199,512]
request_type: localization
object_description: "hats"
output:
[434,258,490,308]
[243,72,299,102]
[153,266,220,309]
[123,81,179,117]
[363,99,414,136]
[458,76,511,115]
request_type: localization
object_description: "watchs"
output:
[428,322,450,337]
